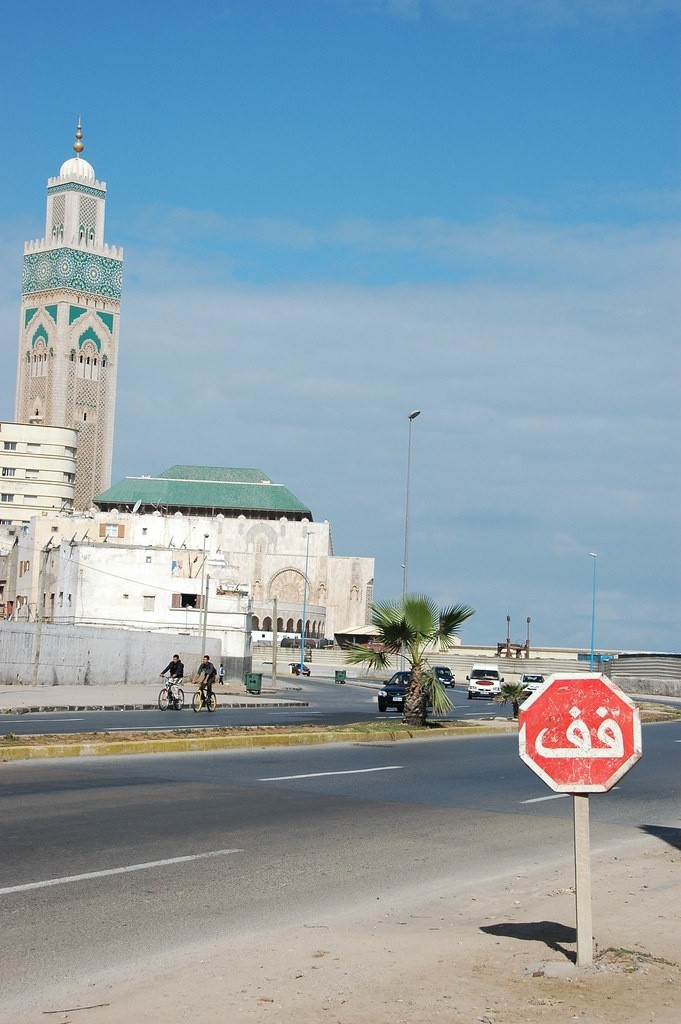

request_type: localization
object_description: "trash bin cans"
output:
[335,669,347,684]
[243,671,263,695]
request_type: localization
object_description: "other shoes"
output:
[175,697,179,701]
[205,695,212,701]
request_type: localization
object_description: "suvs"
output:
[430,666,455,689]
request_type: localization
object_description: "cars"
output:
[288,663,311,677]
[518,673,545,694]
[378,670,436,712]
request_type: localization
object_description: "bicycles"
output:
[191,681,217,713]
[158,675,184,711]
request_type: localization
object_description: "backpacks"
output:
[220,668,224,674]
[208,663,217,675]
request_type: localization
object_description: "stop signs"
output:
[517,673,644,795]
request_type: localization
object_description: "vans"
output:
[466,663,504,700]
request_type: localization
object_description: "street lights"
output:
[199,532,210,636]
[400,409,421,671]
[299,530,316,676]
[589,552,597,673]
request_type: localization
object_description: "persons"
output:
[159,654,183,700]
[192,655,215,707]
[219,664,225,685]
